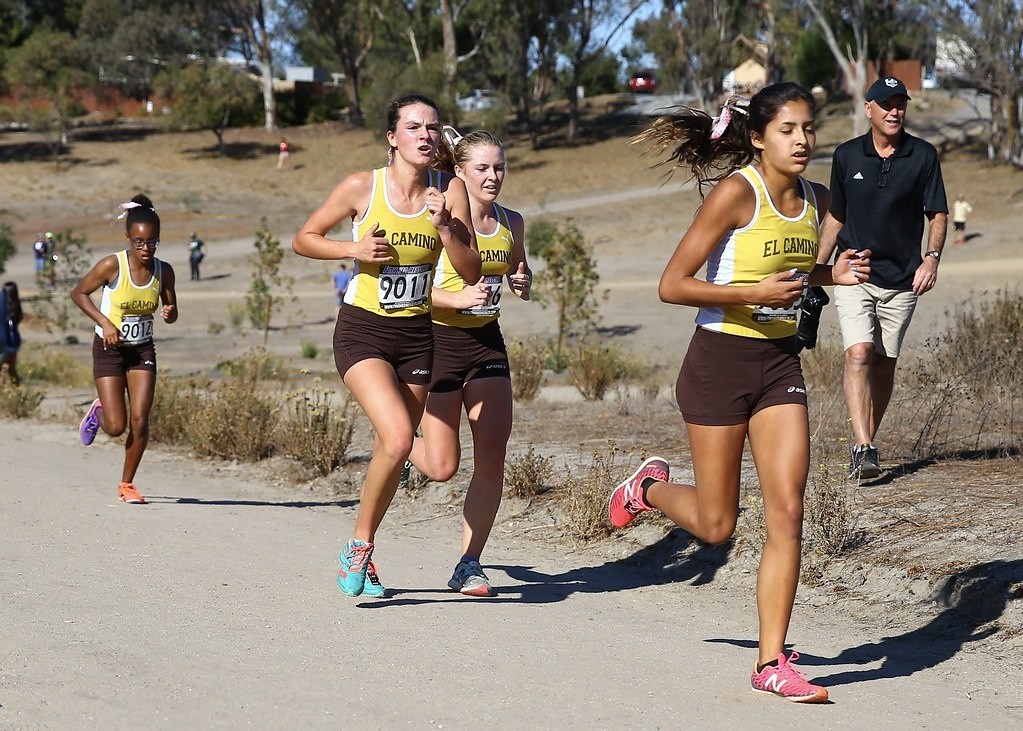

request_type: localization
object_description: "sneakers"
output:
[119,481,145,503]
[398,430,419,483]
[360,558,386,598]
[848,443,882,479]
[608,456,669,527]
[750,651,828,703]
[337,538,374,597]
[448,561,492,596]
[79,398,102,446]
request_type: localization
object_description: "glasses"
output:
[128,235,161,249]
[878,157,891,187]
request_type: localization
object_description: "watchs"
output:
[925,251,940,263]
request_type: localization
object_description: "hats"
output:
[867,76,912,103]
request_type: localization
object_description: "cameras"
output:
[796,286,830,355]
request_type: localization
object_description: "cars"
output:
[626,72,659,93]
[455,89,505,112]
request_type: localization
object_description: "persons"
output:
[291,94,484,598]
[71,194,179,502]
[187,231,205,280]
[333,262,352,320]
[407,130,533,594]
[0,281,24,386]
[815,76,949,480]
[951,194,973,244]
[275,136,290,170]
[609,82,870,702]
[33,231,56,286]
